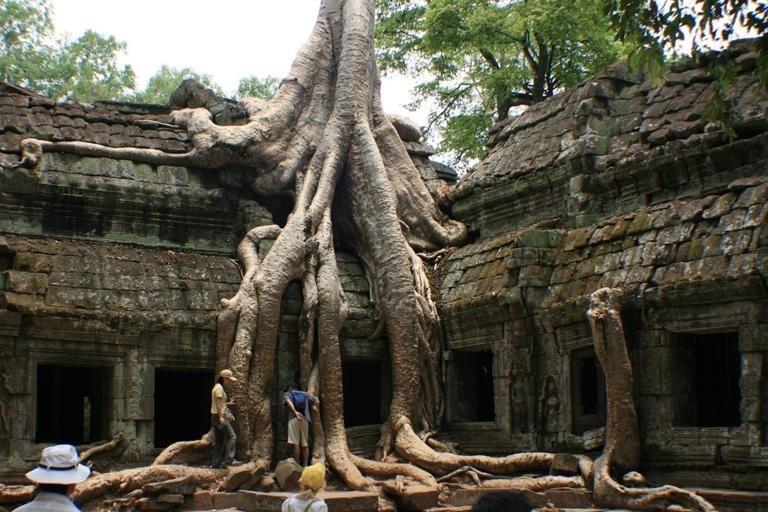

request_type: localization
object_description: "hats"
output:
[25,444,91,485]
[301,462,326,489]
[219,369,238,381]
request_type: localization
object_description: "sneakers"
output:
[219,460,243,468]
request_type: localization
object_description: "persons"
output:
[282,384,321,467]
[470,491,534,512]
[209,369,243,468]
[12,445,91,512]
[281,462,328,511]
